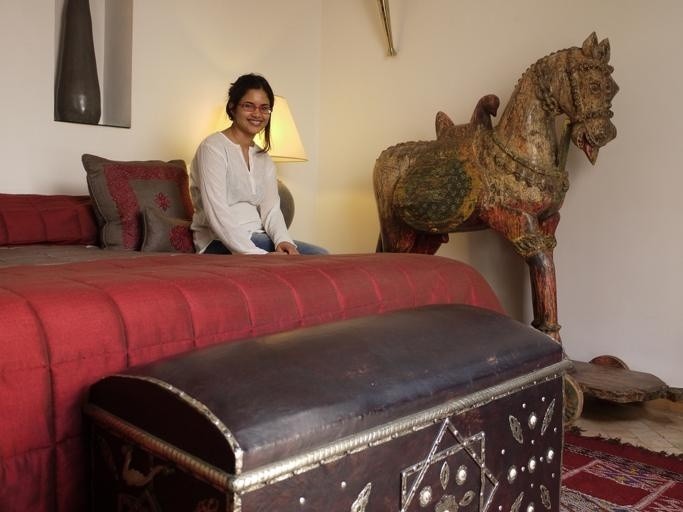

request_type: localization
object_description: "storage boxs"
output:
[81,303,574,512]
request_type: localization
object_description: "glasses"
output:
[238,102,270,114]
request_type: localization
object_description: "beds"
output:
[0,194,511,511]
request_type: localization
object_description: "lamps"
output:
[218,94,309,235]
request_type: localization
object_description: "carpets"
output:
[560,424,683,512]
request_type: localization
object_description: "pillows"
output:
[139,205,196,253]
[81,153,193,252]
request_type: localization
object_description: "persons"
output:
[188,73,329,256]
